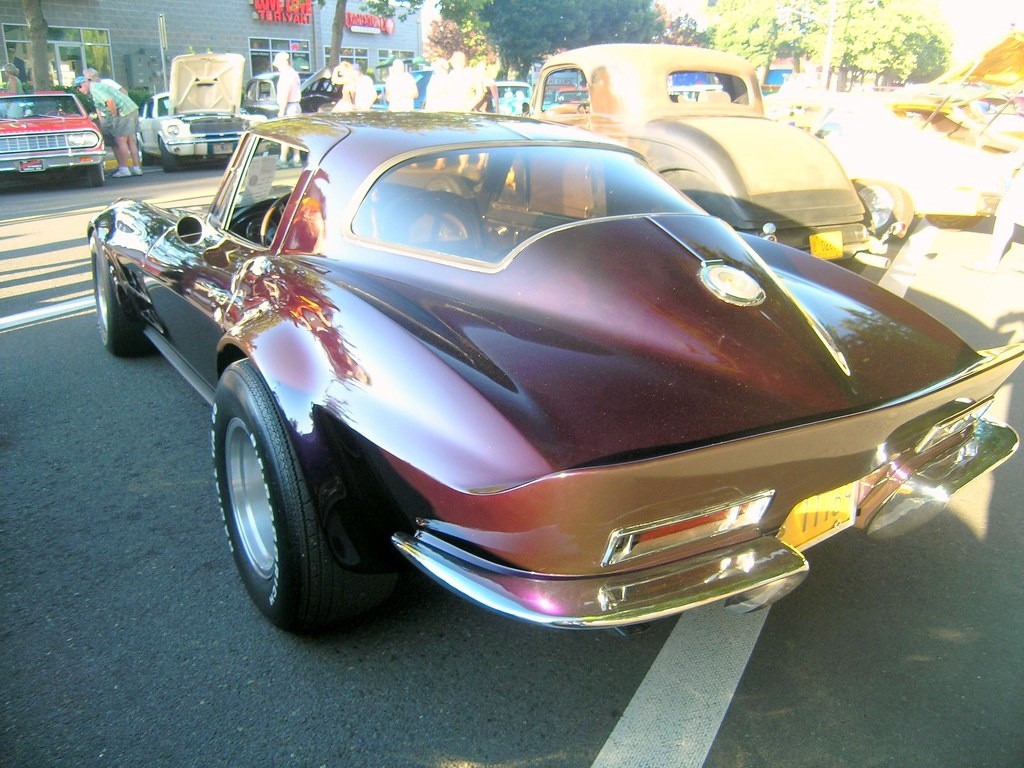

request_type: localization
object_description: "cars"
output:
[0,90,104,188]
[242,71,345,120]
[133,52,266,173]
[780,31,1024,243]
[364,67,535,115]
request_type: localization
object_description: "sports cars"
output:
[519,42,867,264]
[87,112,1024,639]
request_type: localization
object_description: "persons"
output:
[329,49,499,115]
[1,62,27,119]
[961,162,1024,270]
[72,76,144,179]
[83,67,131,177]
[272,51,304,170]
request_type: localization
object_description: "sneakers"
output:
[113,167,131,178]
[131,167,143,176]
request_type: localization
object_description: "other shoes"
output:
[107,168,119,176]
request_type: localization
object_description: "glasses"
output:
[77,86,82,90]
[88,79,92,81]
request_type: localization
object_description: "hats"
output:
[0,66,5,71]
[73,77,87,88]
[272,51,289,65]
